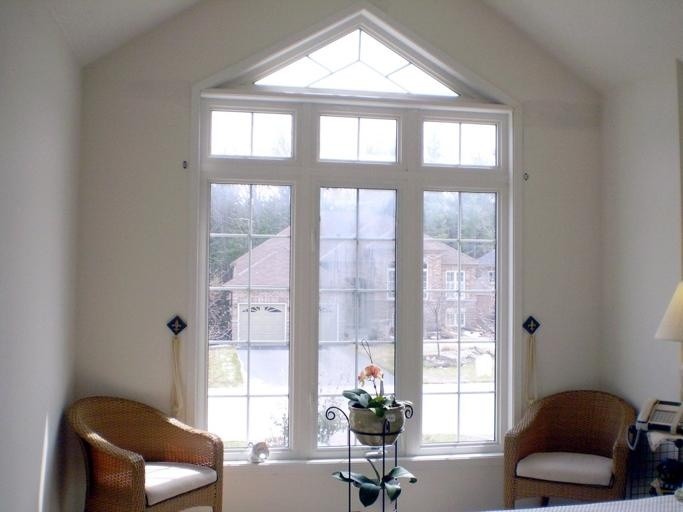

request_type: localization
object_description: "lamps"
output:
[652,282,682,341]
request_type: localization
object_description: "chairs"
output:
[504,390,638,510]
[67,395,224,512]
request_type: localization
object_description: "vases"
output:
[348,401,404,445]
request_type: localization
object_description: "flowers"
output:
[340,364,414,418]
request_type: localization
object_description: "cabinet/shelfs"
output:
[325,405,413,512]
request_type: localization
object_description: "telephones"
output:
[635,398,683,435]
